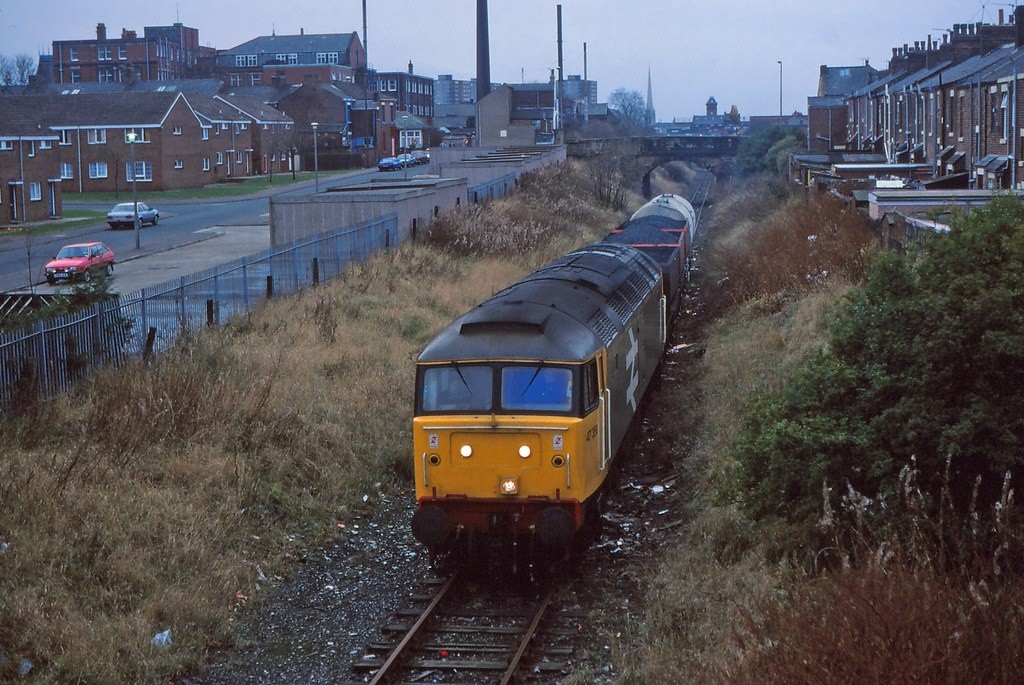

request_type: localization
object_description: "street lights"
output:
[556,66,562,125]
[126,132,141,251]
[401,115,408,180]
[311,122,320,193]
[777,60,784,120]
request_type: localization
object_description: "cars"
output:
[44,242,116,284]
[378,149,431,171]
[106,202,160,229]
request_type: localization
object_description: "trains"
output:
[409,190,698,588]
